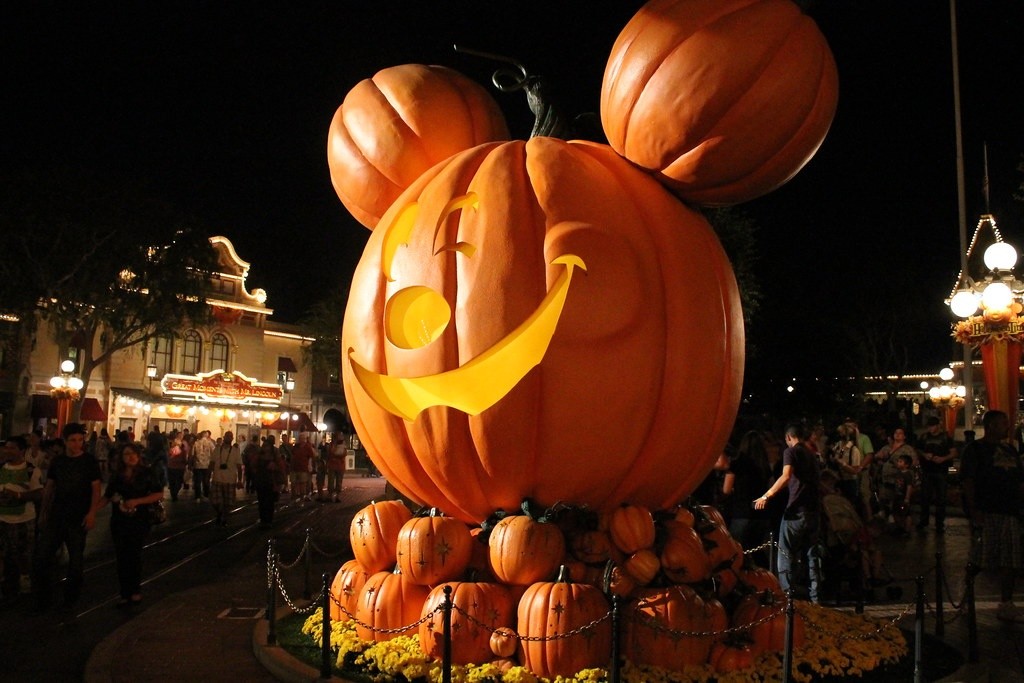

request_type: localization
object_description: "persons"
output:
[239,434,347,528]
[685,417,956,607]
[957,409,1024,623]
[363,462,380,477]
[0,423,243,616]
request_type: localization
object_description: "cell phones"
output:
[751,501,768,510]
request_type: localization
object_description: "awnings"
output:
[262,411,318,431]
[111,386,301,412]
[30,394,108,422]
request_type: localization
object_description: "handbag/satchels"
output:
[149,463,167,523]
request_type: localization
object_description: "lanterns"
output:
[326,0,837,680]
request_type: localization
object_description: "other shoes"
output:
[215,520,223,529]
[196,497,200,503]
[900,531,911,540]
[0,576,5,581]
[915,519,929,530]
[20,575,31,590]
[130,593,141,605]
[996,599,1024,622]
[314,498,325,503]
[223,520,229,527]
[261,522,272,528]
[202,495,209,503]
[936,526,946,535]
[335,497,341,503]
[325,497,332,502]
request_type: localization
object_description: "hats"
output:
[926,416,940,426]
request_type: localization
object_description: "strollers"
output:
[819,487,901,602]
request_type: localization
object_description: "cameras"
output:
[220,463,228,469]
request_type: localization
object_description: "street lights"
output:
[285,375,295,443]
[49,361,83,440]
[145,358,157,432]
[929,368,966,434]
[950,242,1024,435]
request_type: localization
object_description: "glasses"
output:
[839,447,844,459]
[123,451,139,457]
